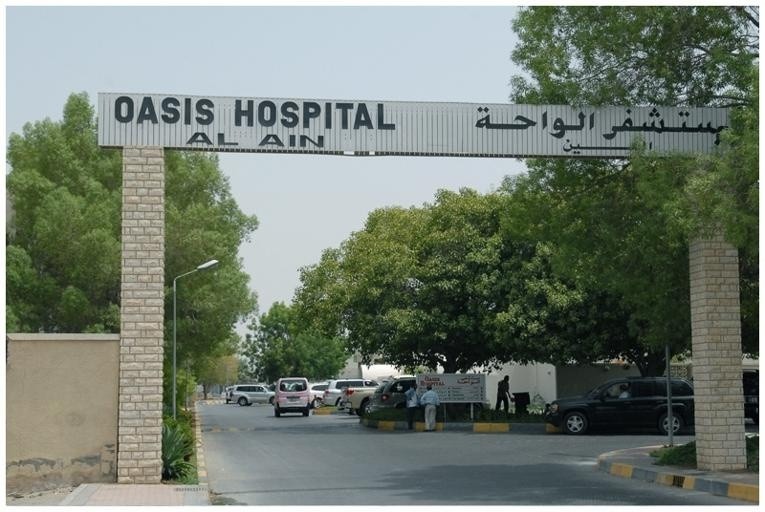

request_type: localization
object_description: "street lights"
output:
[172,259,220,420]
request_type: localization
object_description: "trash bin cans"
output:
[512,392,530,418]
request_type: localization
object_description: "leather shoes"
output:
[424,429,435,432]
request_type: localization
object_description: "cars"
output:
[545,376,694,435]
[225,384,275,406]
[742,368,759,431]
[310,375,417,416]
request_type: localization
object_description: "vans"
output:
[274,377,310,417]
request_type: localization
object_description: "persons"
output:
[618,384,630,399]
[496,376,510,412]
[404,383,420,430]
[419,384,440,432]
